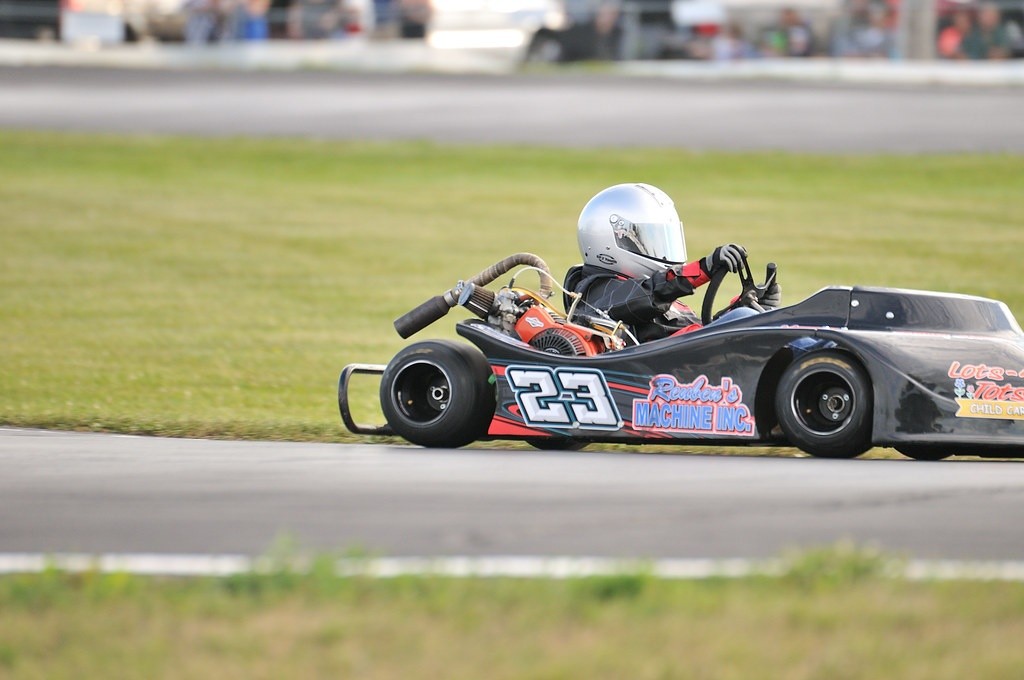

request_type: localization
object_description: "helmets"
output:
[578,184,687,280]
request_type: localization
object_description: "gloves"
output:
[753,283,781,306]
[704,243,748,273]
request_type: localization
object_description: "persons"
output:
[124,0,437,46]
[523,1,1024,60]
[562,182,780,347]
